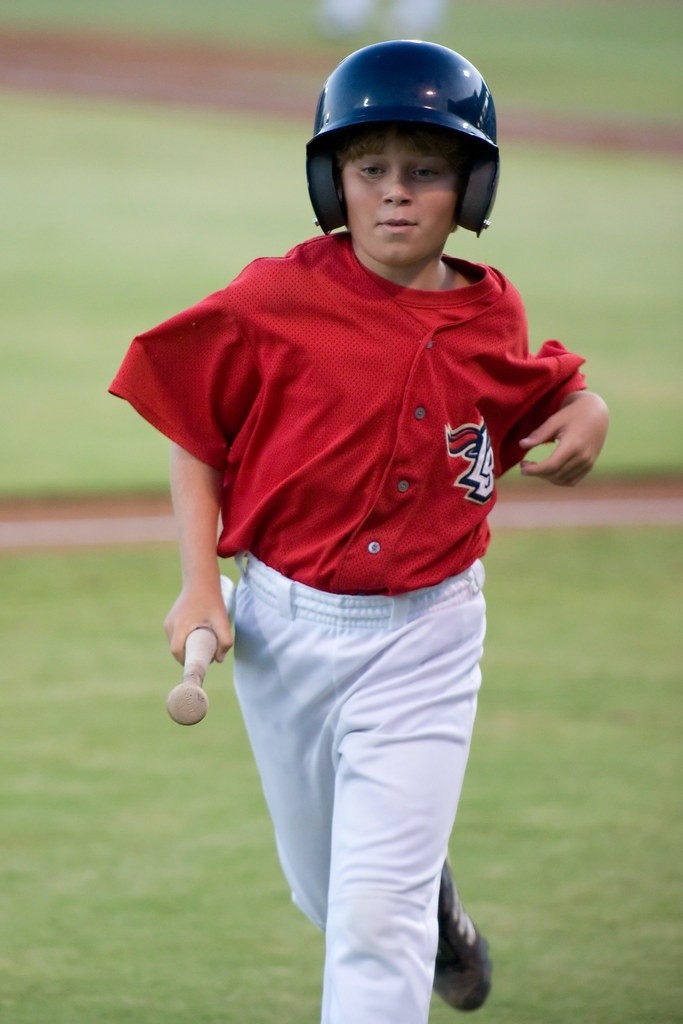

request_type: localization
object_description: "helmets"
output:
[306,39,500,239]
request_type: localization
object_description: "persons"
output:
[108,41,610,1024]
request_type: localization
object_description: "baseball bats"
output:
[166,573,235,728]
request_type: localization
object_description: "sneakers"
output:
[433,853,493,1011]
